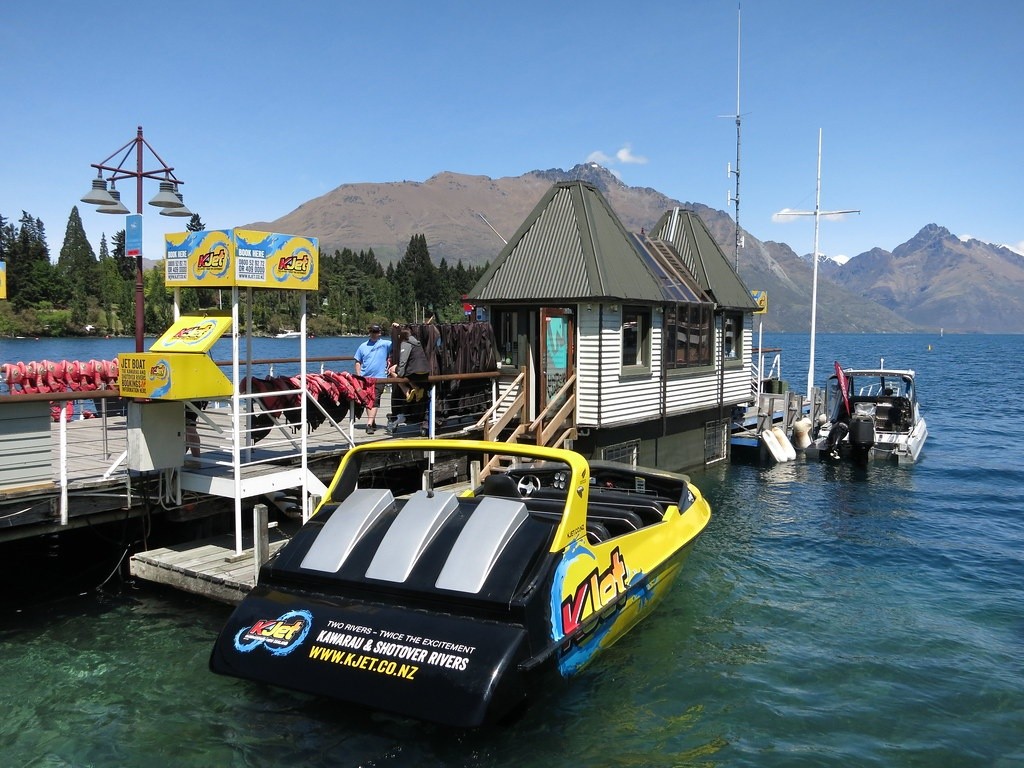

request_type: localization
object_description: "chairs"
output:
[482,475,521,498]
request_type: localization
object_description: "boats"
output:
[271,329,309,339]
[337,332,369,338]
[208,438,711,731]
[800,357,929,466]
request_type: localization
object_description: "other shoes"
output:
[406,389,414,403]
[416,388,424,402]
[373,422,377,431]
[365,425,374,434]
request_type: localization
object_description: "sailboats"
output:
[729,126,829,465]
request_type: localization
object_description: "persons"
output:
[390,327,429,403]
[353,322,400,435]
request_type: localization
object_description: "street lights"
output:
[81,125,194,354]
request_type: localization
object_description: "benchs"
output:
[476,494,642,529]
[533,513,610,543]
[530,489,664,523]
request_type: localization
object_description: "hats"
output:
[368,324,381,333]
